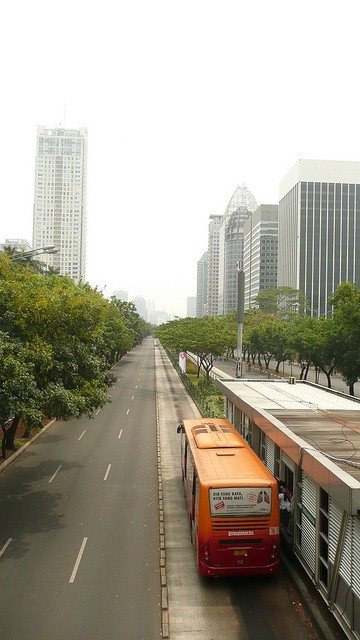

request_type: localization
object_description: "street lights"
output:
[9,245,56,257]
[11,249,61,262]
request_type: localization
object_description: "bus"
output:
[177,417,281,581]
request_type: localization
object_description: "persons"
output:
[277,480,294,535]
